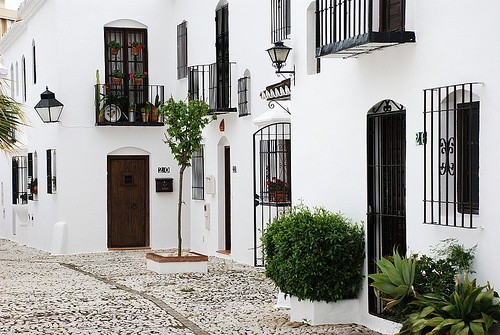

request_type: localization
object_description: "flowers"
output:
[266,177,288,193]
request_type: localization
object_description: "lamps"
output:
[264,40,295,86]
[34,85,65,124]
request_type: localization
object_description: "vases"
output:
[270,192,285,202]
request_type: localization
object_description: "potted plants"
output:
[96,39,163,124]
[248,197,365,326]
[368,237,499,335]
